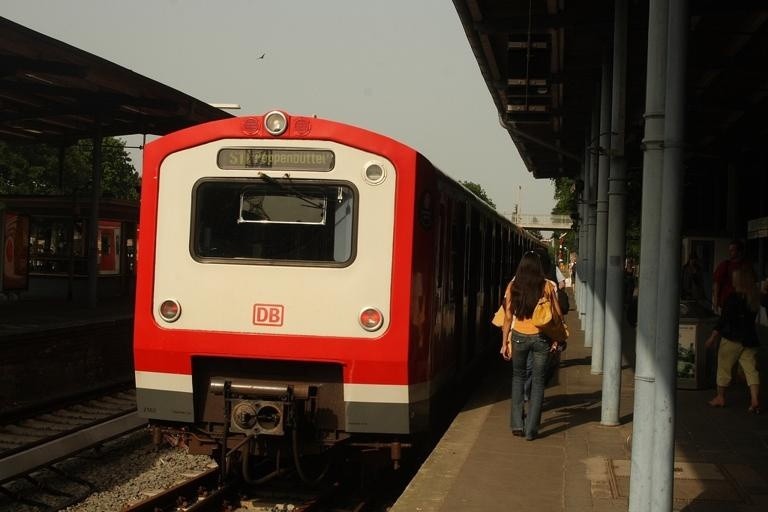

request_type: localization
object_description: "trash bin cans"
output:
[676,300,722,390]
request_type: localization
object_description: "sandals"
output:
[746,405,761,414]
[708,399,726,407]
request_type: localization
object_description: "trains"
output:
[125,112,554,499]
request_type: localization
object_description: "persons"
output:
[622,237,766,417]
[499,245,566,403]
[498,250,563,442]
[569,261,576,292]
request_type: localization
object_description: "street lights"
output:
[516,185,522,221]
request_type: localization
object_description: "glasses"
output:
[524,251,540,259]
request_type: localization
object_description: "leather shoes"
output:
[513,431,525,436]
[527,434,540,440]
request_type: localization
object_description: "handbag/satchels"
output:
[536,284,568,344]
